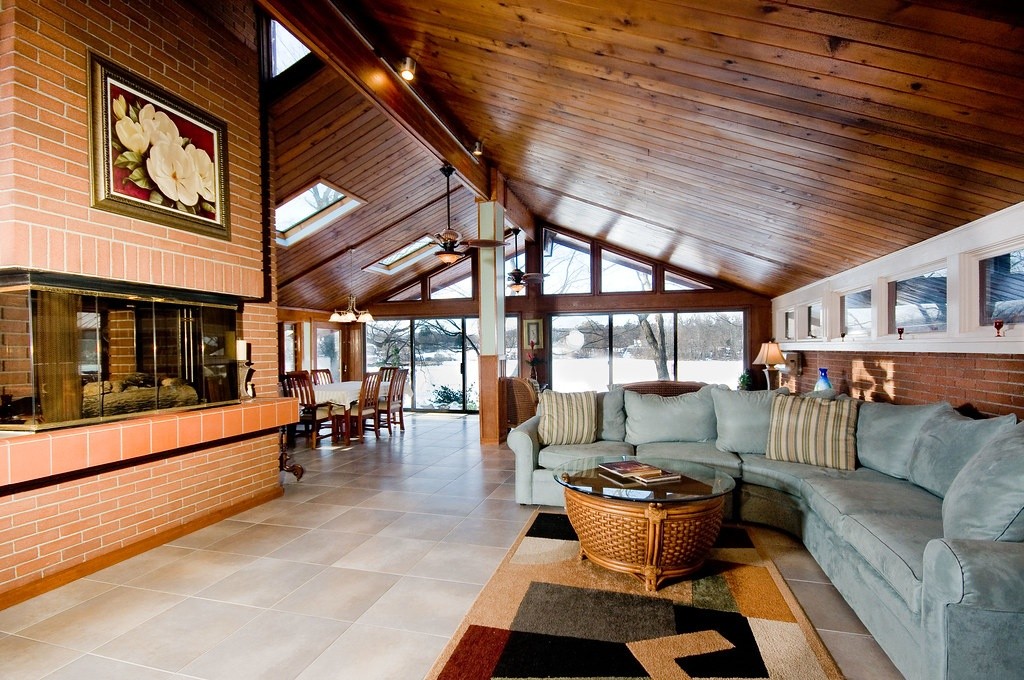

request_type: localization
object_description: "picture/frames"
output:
[523,319,544,349]
[86,49,232,242]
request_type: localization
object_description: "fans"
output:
[505,229,550,283]
[384,165,510,248]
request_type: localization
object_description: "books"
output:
[598,460,681,488]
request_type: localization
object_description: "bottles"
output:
[813,368,834,391]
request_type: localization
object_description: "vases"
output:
[814,367,833,391]
[529,365,537,381]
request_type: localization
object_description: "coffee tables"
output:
[552,457,736,592]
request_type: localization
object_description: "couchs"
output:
[506,384,1024,680]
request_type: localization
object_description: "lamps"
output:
[508,283,527,293]
[328,249,375,323]
[434,246,466,266]
[466,142,483,155]
[752,341,787,390]
[399,57,416,81]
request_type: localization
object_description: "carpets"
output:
[422,509,846,679]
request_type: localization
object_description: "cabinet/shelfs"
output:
[770,201,1024,355]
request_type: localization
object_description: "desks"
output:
[284,380,402,447]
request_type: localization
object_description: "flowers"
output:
[524,340,540,367]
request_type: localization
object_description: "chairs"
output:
[280,366,409,450]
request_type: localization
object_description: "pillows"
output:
[538,389,596,446]
[766,392,857,472]
